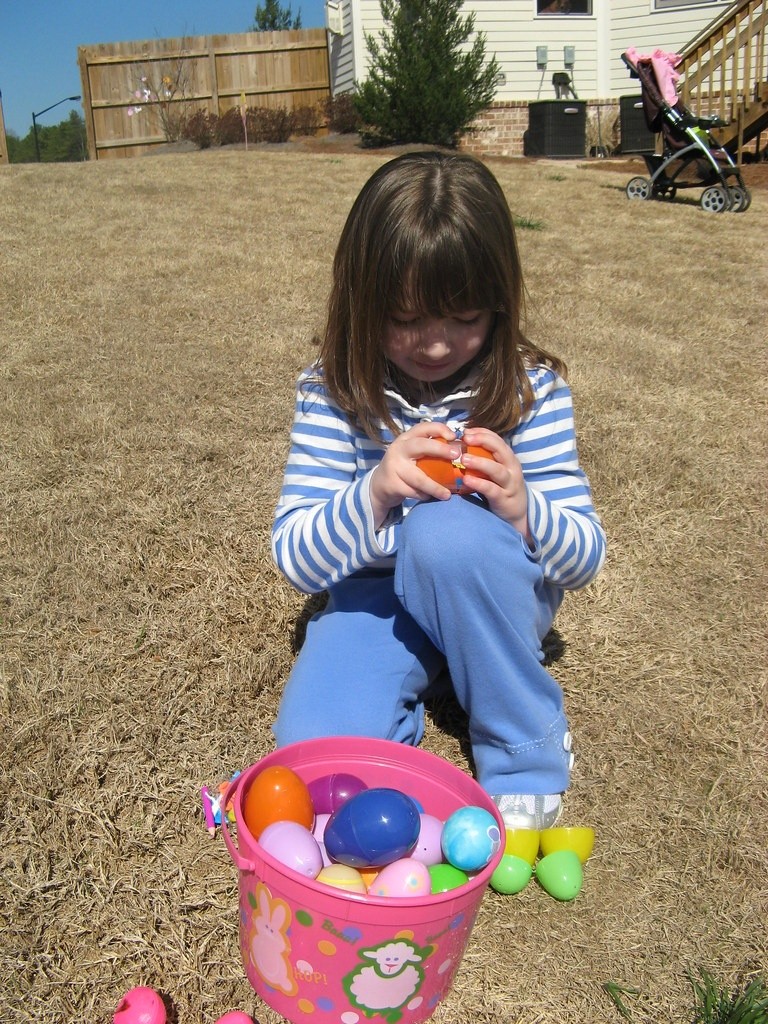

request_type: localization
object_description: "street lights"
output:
[31,95,82,162]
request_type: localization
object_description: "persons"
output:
[271,152,607,831]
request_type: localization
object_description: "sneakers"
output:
[489,793,564,830]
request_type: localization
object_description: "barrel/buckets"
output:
[220,740,507,1024]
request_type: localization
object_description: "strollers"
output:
[620,47,753,214]
